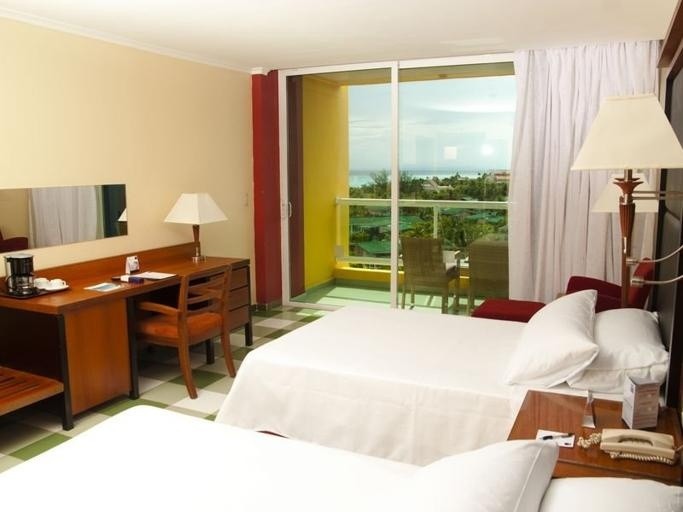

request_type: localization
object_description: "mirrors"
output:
[0,184,128,254]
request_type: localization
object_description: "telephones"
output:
[600,428,676,465]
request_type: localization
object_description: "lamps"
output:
[590,173,659,213]
[117,207,127,222]
[163,192,228,262]
[571,92,683,309]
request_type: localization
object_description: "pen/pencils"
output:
[539,432,573,439]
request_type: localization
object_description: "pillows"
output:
[539,476,683,512]
[407,439,559,512]
[569,308,670,393]
[506,289,599,385]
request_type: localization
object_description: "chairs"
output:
[467,237,508,310]
[470,257,652,322]
[136,264,235,399]
[398,236,465,314]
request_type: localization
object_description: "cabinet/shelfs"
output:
[0,241,252,430]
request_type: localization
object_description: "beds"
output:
[0,405,683,512]
[214,300,667,466]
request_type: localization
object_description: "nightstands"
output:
[506,389,683,487]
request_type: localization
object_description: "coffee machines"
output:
[4,253,36,297]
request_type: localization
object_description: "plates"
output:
[46,285,70,293]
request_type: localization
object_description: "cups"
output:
[34,278,66,290]
